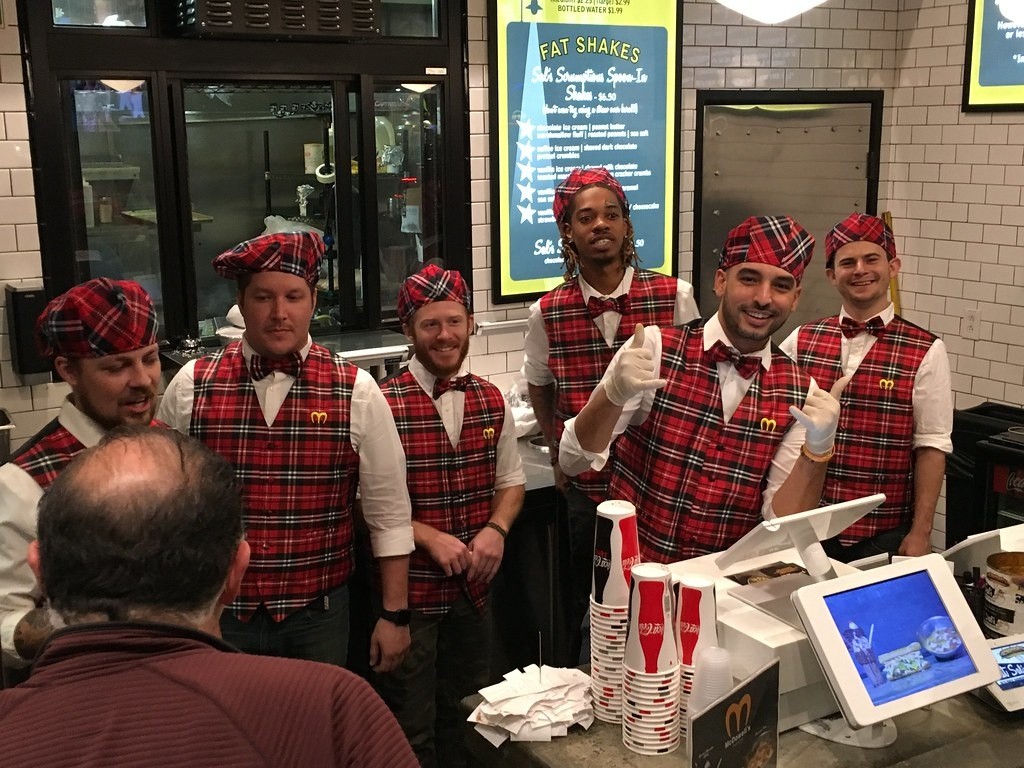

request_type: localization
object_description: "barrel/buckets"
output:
[375,113,396,173]
[983,551,1024,639]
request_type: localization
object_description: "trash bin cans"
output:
[946,402,1024,549]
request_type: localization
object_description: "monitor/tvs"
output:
[794,552,1002,728]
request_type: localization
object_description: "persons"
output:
[558,217,850,665]
[524,168,703,672]
[378,263,527,768]
[776,212,953,564]
[155,232,415,677]
[0,422,422,768]
[0,276,160,689]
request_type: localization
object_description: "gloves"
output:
[789,377,851,455]
[603,323,667,408]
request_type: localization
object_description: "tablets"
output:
[978,634,1024,713]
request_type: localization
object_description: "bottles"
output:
[83,177,95,227]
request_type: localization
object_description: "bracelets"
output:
[800,445,830,465]
[803,444,836,463]
[550,456,559,467]
[486,522,507,539]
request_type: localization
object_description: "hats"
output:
[395,263,472,324]
[35,277,158,359]
[825,212,896,268]
[717,214,815,287]
[211,230,324,289]
[552,167,626,231]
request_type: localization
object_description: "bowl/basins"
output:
[916,616,963,659]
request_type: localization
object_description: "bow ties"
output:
[706,339,762,379]
[841,316,885,340]
[433,374,472,399]
[586,293,631,319]
[250,350,303,381]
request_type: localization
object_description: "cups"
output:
[589,499,734,761]
[852,630,886,688]
[99,195,113,223]
[303,143,324,174]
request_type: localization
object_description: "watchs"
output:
[379,608,412,625]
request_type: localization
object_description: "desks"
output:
[122,208,214,275]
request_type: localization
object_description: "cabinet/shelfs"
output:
[263,128,410,305]
